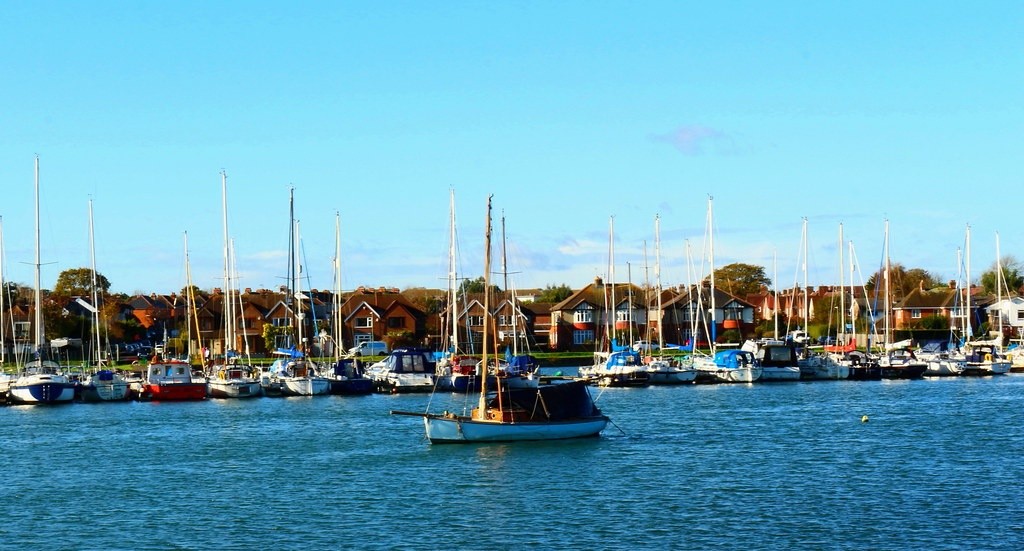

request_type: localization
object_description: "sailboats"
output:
[423,192,613,443]
[0,153,1024,406]
